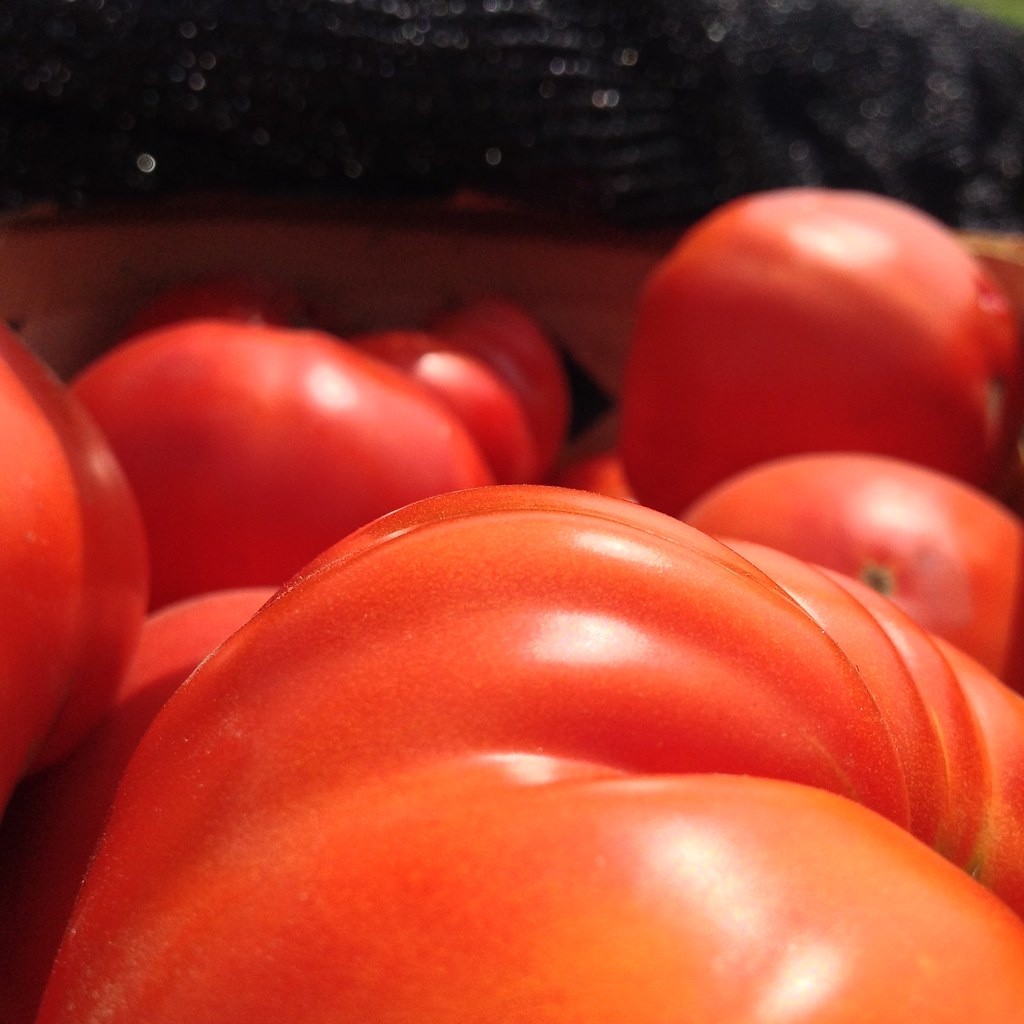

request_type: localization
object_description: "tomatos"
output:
[0,186,1024,1024]
